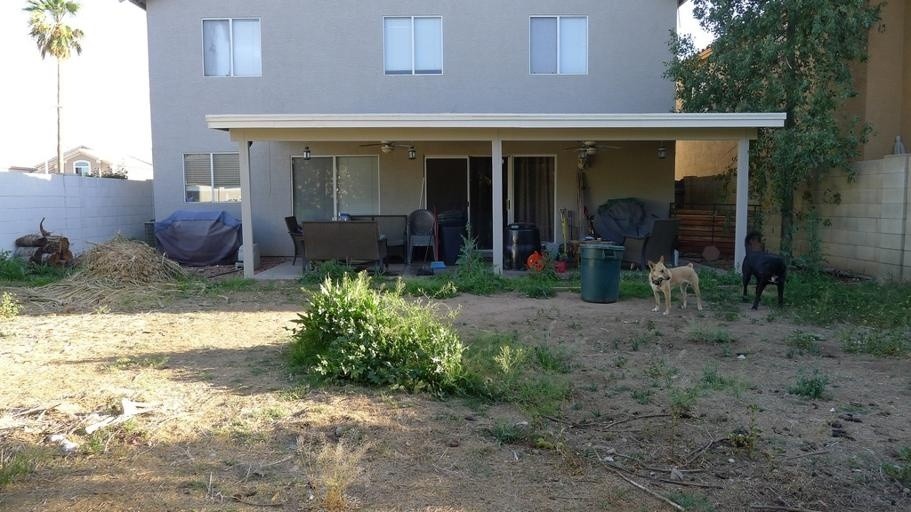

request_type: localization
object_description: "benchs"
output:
[300,217,390,273]
[350,214,409,259]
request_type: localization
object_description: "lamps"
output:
[657,139,669,160]
[303,145,312,161]
[407,146,417,161]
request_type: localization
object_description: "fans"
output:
[561,140,623,156]
[359,141,413,154]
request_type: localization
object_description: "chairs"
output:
[407,209,440,268]
[285,215,307,267]
[621,218,683,272]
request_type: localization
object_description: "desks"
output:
[566,239,617,267]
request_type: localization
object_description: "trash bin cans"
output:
[580,243,625,303]
[436,214,468,266]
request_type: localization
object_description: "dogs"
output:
[647,255,703,315]
[742,231,787,310]
